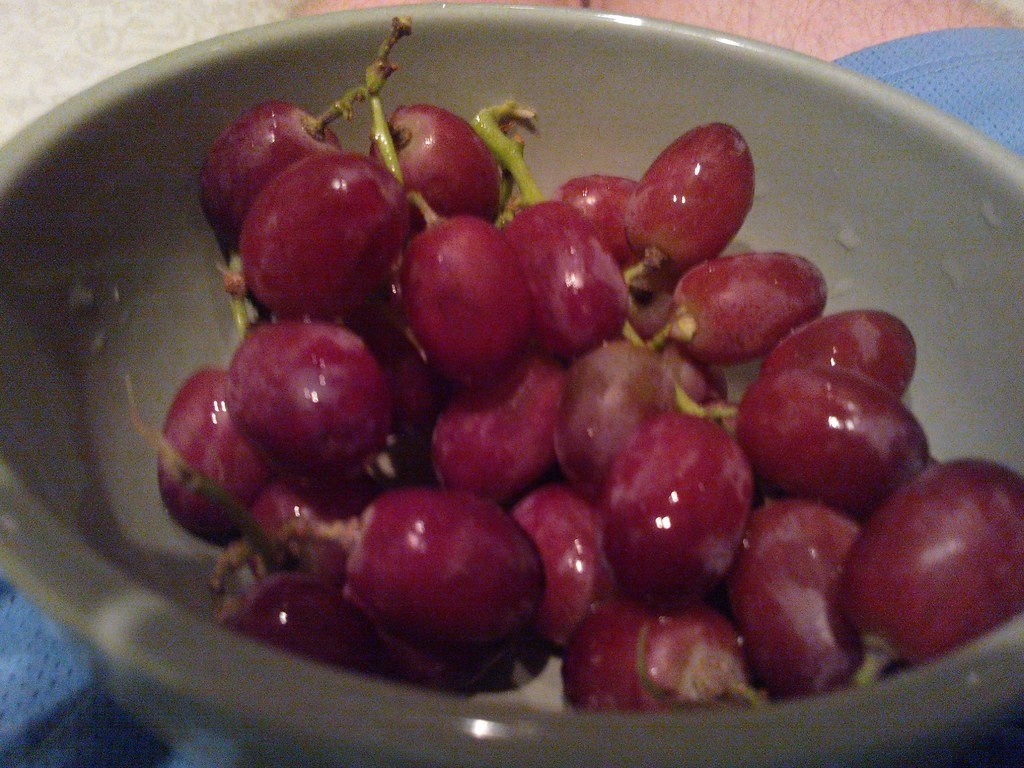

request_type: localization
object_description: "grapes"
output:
[157,101,1024,715]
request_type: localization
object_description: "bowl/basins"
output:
[0,0,1024,768]
